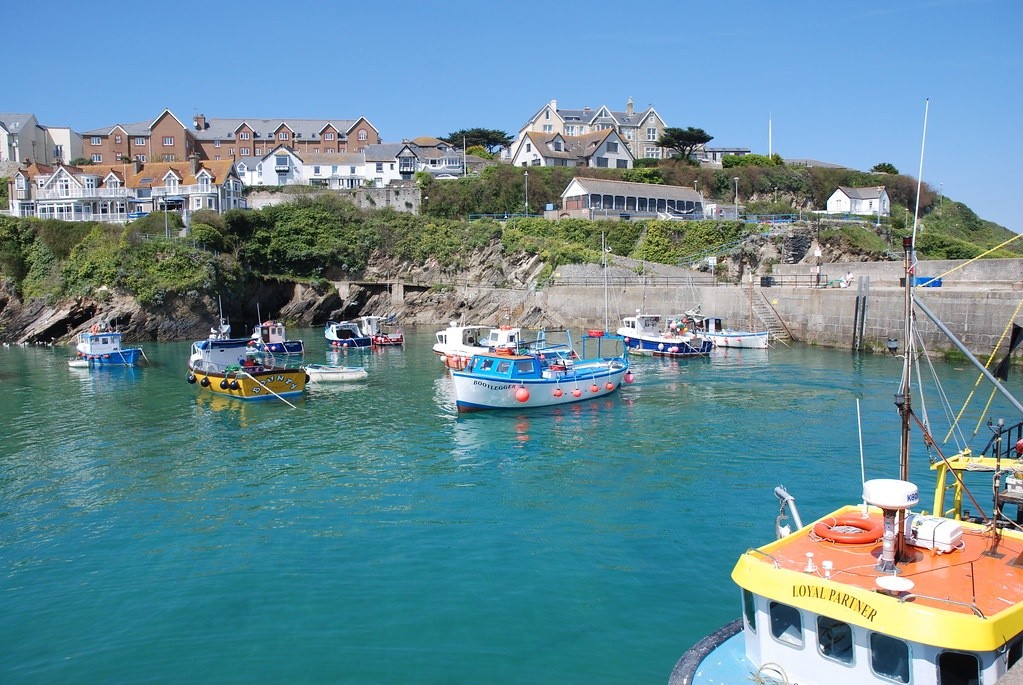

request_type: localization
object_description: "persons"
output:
[817,274,820,287]
[846,271,854,286]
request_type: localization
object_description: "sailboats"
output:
[665,96,1023,684]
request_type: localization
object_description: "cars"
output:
[434,174,458,180]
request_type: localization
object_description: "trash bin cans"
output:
[761,276,772,287]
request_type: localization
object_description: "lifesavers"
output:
[814,512,886,544]
[496,348,512,355]
[304,374,311,384]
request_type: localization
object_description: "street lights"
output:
[940,182,943,208]
[733,177,739,221]
[523,170,529,214]
[693,180,698,191]
[905,208,908,229]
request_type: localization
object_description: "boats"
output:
[678,272,770,349]
[447,230,636,413]
[351,270,404,346]
[432,280,522,372]
[185,294,311,401]
[250,301,305,356]
[324,321,374,349]
[616,272,713,356]
[67,311,144,368]
[301,364,369,382]
